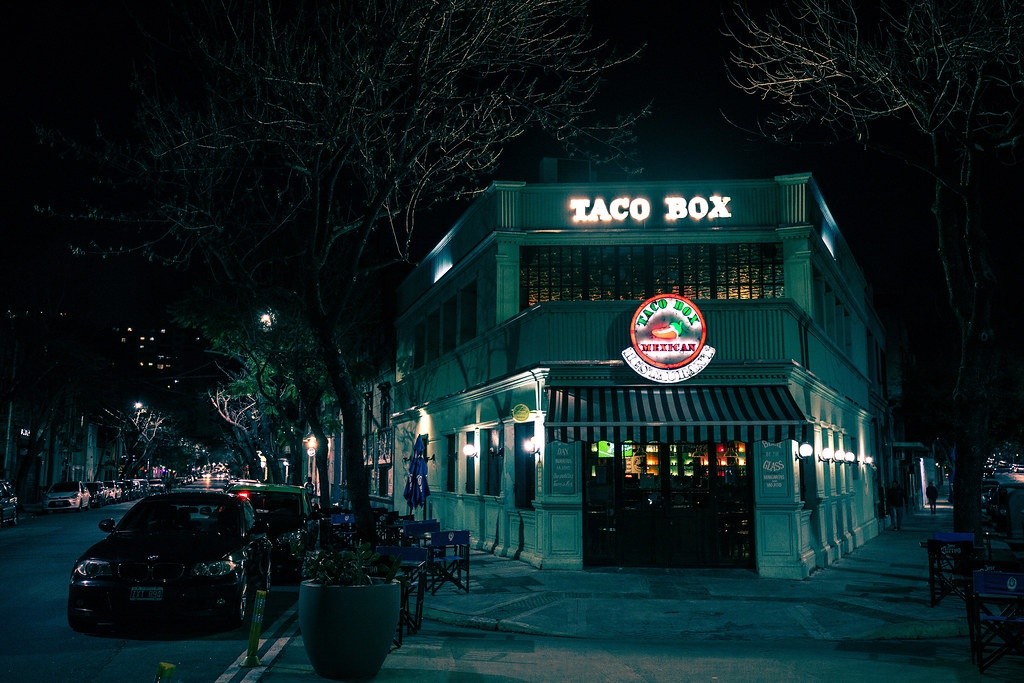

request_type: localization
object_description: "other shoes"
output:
[893,527,902,531]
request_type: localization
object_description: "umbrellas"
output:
[404,434,431,515]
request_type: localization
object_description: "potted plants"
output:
[291,540,412,682]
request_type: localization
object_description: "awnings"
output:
[544,384,810,444]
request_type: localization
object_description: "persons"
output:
[927,483,939,515]
[889,480,905,531]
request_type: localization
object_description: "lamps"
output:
[524,442,540,458]
[794,441,813,464]
[818,448,872,466]
[463,444,478,458]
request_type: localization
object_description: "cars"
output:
[0,479,19,527]
[981,477,1024,545]
[84,474,197,507]
[42,481,92,513]
[67,490,275,632]
[201,471,316,528]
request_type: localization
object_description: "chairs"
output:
[329,511,471,646]
[141,504,172,527]
[921,531,1024,671]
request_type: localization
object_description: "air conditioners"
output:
[896,448,905,459]
